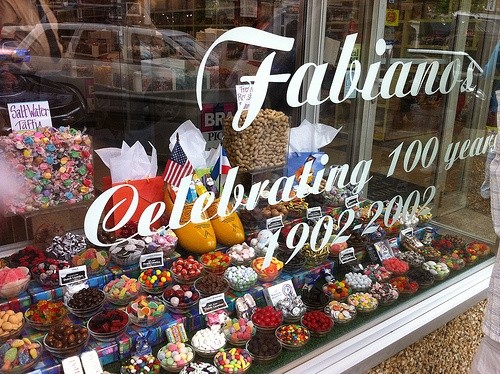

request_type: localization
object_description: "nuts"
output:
[223,108,289,171]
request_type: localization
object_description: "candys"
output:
[0,181,490,374]
[0,126,93,214]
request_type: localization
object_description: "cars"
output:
[0,65,90,136]
[383,17,499,128]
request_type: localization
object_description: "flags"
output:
[163,142,193,186]
[210,146,231,180]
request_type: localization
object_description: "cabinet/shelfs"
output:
[0,157,498,374]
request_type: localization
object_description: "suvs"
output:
[4,22,229,99]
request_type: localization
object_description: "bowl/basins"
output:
[0,198,491,374]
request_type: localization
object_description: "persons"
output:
[130,0,162,46]
[0,0,62,79]
[248,0,273,32]
